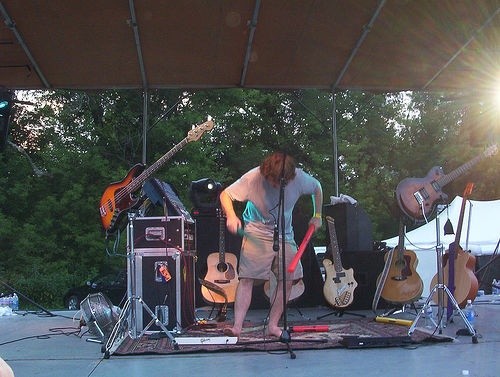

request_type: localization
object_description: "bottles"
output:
[460,370,470,377]
[491,279,500,296]
[424,304,433,328]
[0,292,19,310]
[464,300,474,328]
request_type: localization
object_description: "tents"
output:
[377,196,500,298]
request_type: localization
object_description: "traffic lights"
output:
[0,91,14,153]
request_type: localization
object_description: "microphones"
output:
[272,223,280,251]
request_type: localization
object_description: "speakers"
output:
[193,215,244,310]
[324,201,373,254]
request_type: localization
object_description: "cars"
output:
[64,270,128,311]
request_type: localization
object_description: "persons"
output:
[0,292,18,311]
[220,151,323,338]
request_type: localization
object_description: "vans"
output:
[313,247,327,282]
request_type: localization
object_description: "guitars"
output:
[97,119,216,234]
[375,212,424,306]
[429,181,479,312]
[199,183,242,308]
[394,141,500,223]
[321,214,359,310]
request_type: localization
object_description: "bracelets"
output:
[313,213,322,219]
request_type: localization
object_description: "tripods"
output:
[101,208,180,360]
[316,308,367,319]
[236,154,328,360]
[408,205,479,344]
